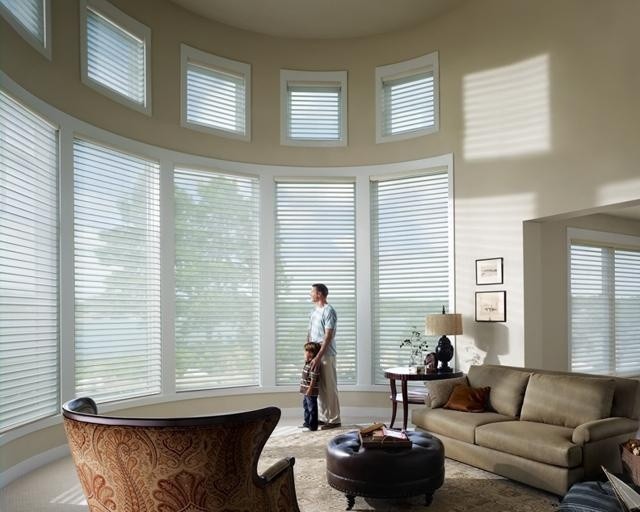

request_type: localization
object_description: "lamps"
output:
[424,305,463,372]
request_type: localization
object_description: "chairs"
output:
[62,397,301,512]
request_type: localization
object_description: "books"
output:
[406,390,429,401]
[355,421,411,448]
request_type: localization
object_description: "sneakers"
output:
[317,420,341,430]
[298,423,313,432]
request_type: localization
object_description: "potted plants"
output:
[400,326,428,372]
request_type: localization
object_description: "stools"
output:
[327,429,444,510]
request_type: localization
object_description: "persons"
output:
[299,342,322,432]
[305,284,341,430]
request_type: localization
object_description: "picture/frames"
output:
[474,290,507,322]
[476,256,504,286]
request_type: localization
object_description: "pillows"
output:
[520,373,617,427]
[444,384,491,413]
[466,364,530,417]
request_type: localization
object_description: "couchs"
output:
[412,364,640,498]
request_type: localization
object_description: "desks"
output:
[383,366,463,431]
